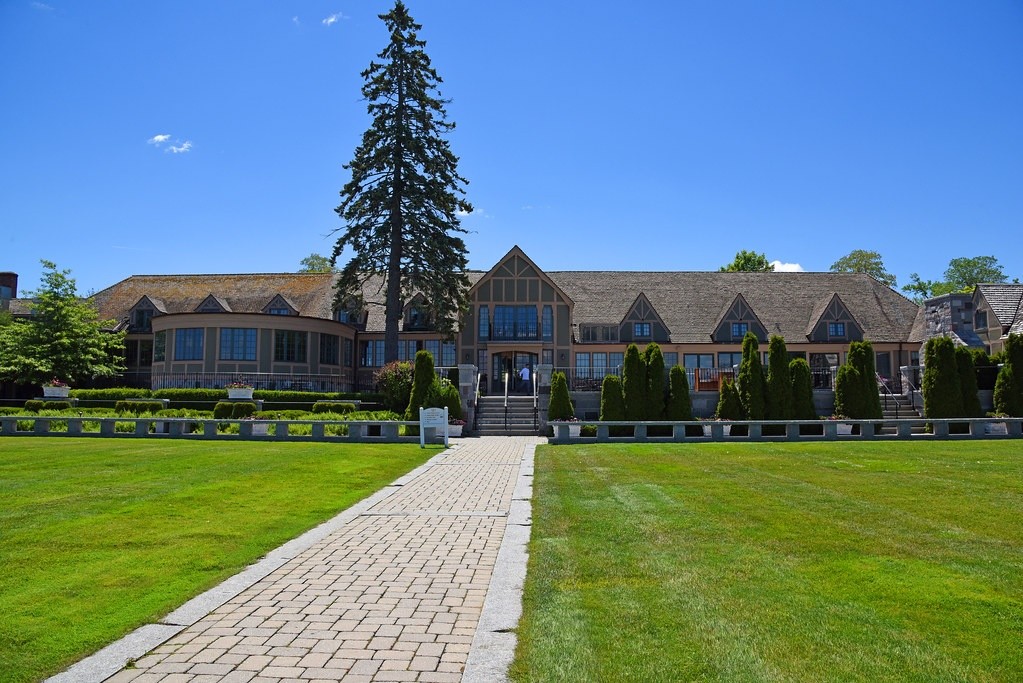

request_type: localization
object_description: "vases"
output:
[42,386,71,397]
[702,425,731,435]
[227,388,254,398]
[552,425,582,437]
[436,425,464,436]
[836,424,853,434]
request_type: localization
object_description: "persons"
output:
[517,364,532,396]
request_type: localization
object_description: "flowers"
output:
[824,413,850,420]
[552,415,581,422]
[694,414,731,421]
[224,381,255,388]
[448,415,466,425]
[42,376,67,387]
